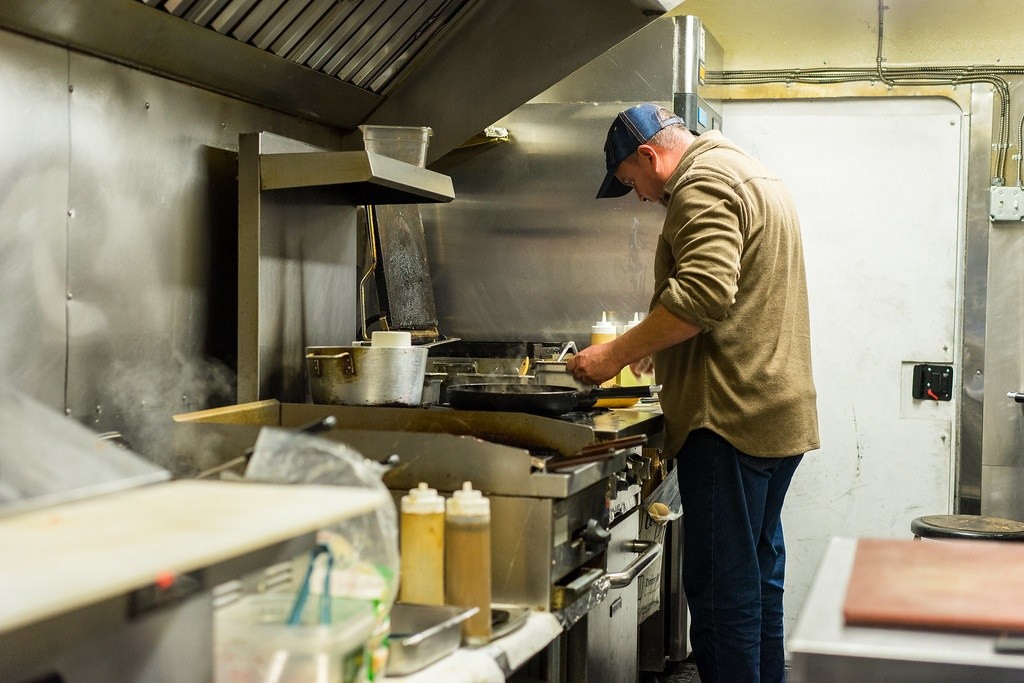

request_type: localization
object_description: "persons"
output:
[565,105,821,683]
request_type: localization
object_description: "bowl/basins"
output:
[357,124,435,169]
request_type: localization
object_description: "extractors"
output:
[0,0,688,166]
[259,150,455,204]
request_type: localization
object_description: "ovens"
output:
[566,440,664,683]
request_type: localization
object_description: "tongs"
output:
[259,542,337,683]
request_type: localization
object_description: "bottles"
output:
[620,309,652,387]
[444,479,492,645]
[397,481,445,608]
[591,306,618,388]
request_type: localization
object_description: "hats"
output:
[595,103,685,200]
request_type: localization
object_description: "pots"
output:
[445,383,664,419]
[302,344,429,407]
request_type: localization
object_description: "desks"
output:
[786,537,1024,683]
